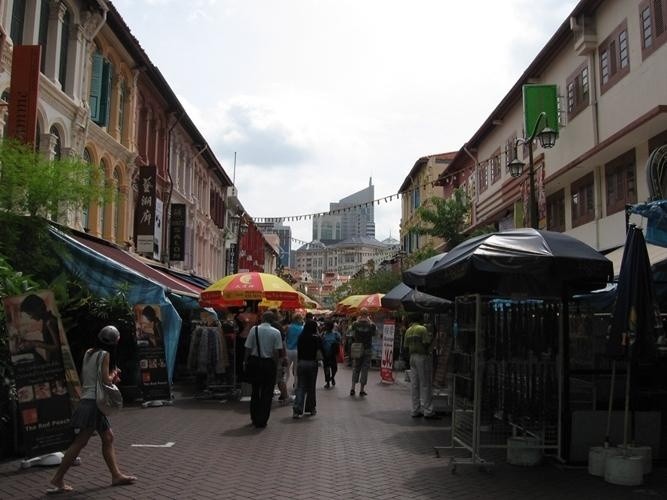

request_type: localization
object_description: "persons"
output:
[268,322,291,402]
[304,312,314,323]
[292,320,324,419]
[320,322,341,389]
[141,305,164,348]
[316,317,326,367]
[344,316,358,368]
[17,292,62,364]
[44,324,136,494]
[403,313,443,420]
[240,310,285,429]
[283,313,305,395]
[398,316,411,371]
[347,307,377,398]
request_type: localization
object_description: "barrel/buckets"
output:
[112,474,137,482]
[47,485,73,493]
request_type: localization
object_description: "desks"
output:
[231,211,248,274]
[507,112,557,229]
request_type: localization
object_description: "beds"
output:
[380,279,455,315]
[347,293,397,314]
[605,221,637,444]
[424,227,615,439]
[400,249,457,303]
[334,294,371,314]
[257,290,320,321]
[198,297,248,308]
[295,300,331,315]
[199,271,305,314]
[605,224,657,444]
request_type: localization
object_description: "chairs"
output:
[293,409,302,418]
[305,406,316,415]
[350,389,355,396]
[412,412,423,418]
[424,414,442,420]
[324,384,329,388]
[278,395,296,405]
[331,377,335,385]
[360,392,367,396]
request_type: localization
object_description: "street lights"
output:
[351,343,364,358]
[96,382,123,416]
[316,350,323,361]
[331,342,340,354]
[336,344,345,364]
[244,357,263,384]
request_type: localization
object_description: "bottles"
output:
[359,307,368,315]
[99,326,121,344]
[306,312,315,319]
